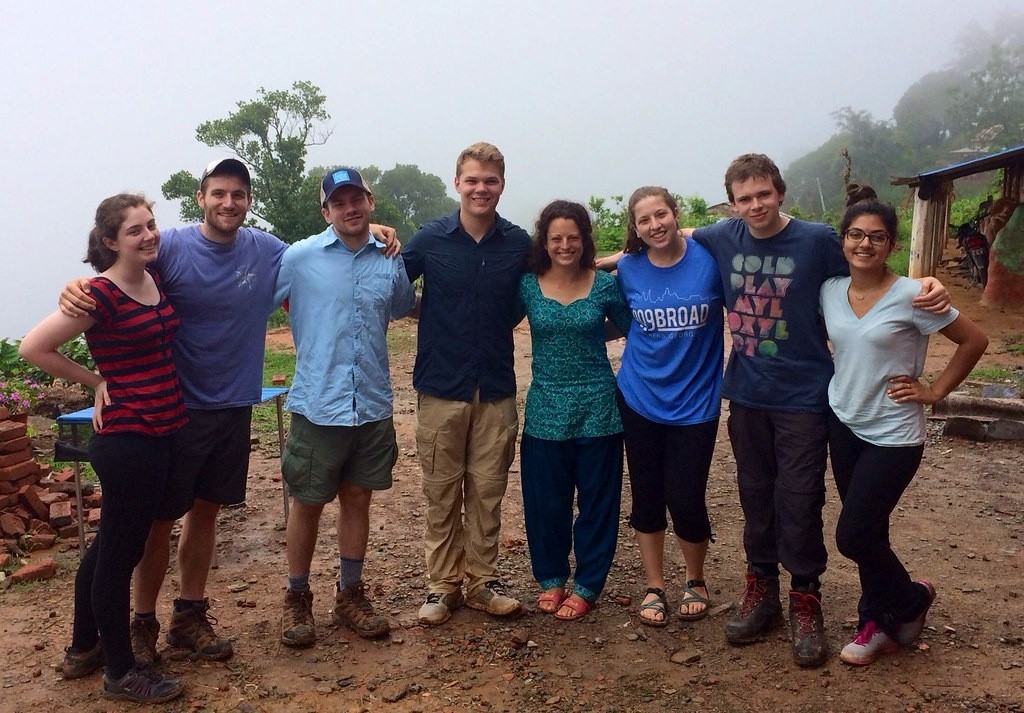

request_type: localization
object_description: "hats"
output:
[319,169,373,208]
[201,157,254,191]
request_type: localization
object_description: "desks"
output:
[56,388,289,562]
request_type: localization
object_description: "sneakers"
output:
[466,581,520,615]
[840,620,899,664]
[130,622,160,666]
[102,669,182,703]
[279,589,317,644]
[332,581,389,636]
[165,603,236,660]
[895,579,936,644]
[418,588,465,624]
[63,635,107,678]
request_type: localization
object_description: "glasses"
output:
[841,227,893,246]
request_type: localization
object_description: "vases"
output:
[8,411,28,425]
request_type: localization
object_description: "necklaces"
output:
[850,270,887,300]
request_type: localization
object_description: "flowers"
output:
[0,380,44,416]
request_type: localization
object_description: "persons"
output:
[515,201,633,620]
[594,155,952,665]
[59,158,402,664]
[601,185,729,628]
[818,185,988,665]
[400,142,541,626]
[18,193,190,704]
[269,170,424,646]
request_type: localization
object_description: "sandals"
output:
[678,579,708,619]
[640,587,668,626]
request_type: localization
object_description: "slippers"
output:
[537,586,565,612]
[555,599,592,620]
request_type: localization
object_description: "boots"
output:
[788,583,827,665]
[724,571,783,643]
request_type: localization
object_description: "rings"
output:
[908,384,911,388]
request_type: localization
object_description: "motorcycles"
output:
[948,201,989,290]
